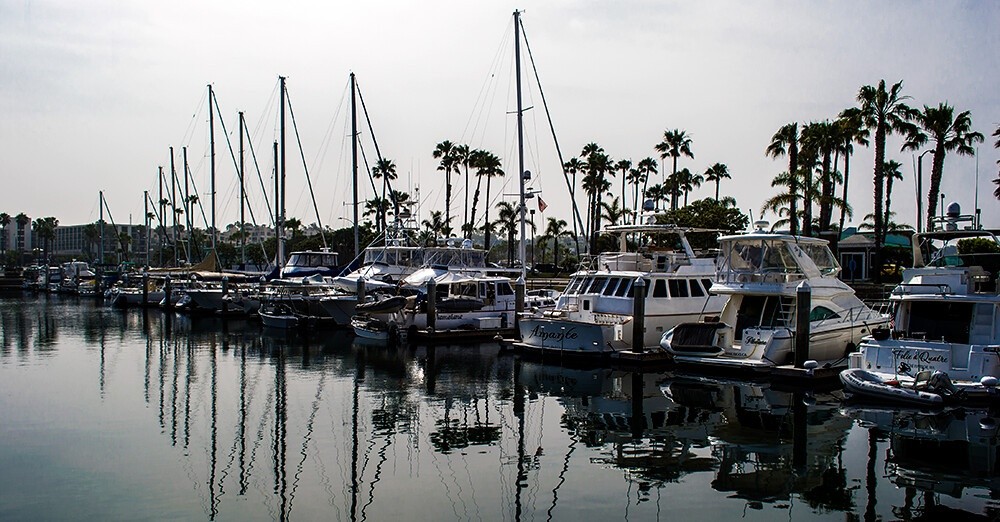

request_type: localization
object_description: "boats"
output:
[845,195,1000,393]
[838,369,956,407]
[659,220,890,372]
[513,224,731,353]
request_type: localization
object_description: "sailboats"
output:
[95,9,585,344]
